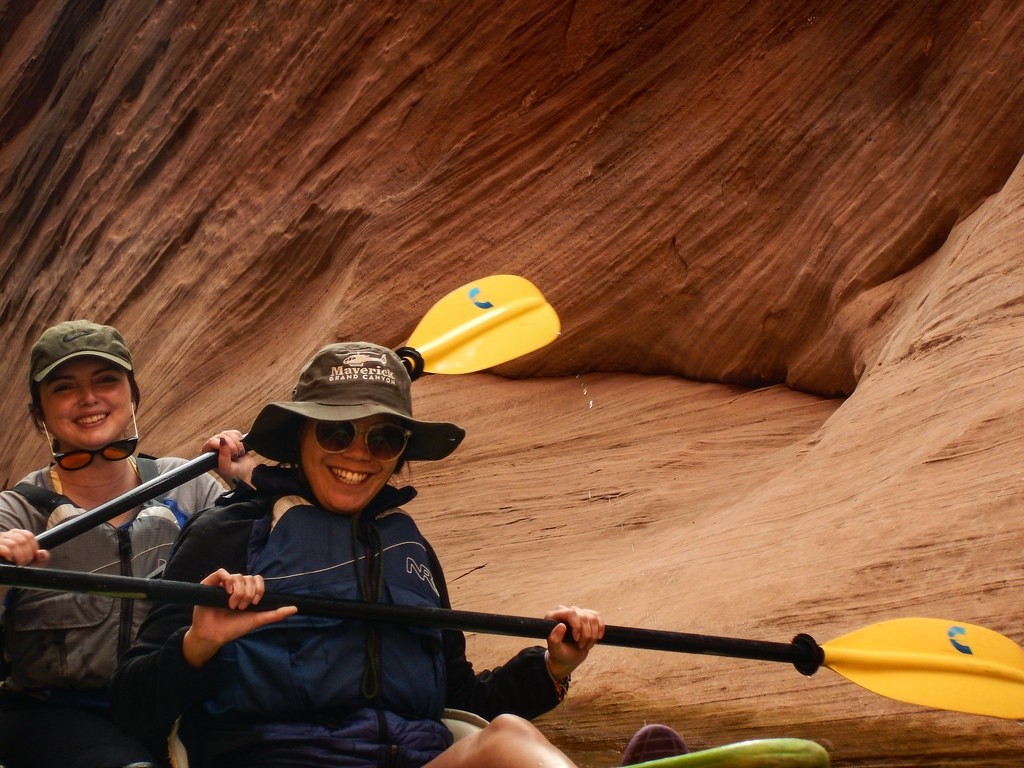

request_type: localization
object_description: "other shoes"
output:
[619,724,692,767]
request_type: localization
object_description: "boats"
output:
[618,735,836,768]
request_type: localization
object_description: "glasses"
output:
[34,402,139,471]
[312,420,413,461]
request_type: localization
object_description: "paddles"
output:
[0,273,562,564]
[0,564,1024,723]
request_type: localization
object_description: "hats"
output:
[29,319,133,401]
[240,341,466,461]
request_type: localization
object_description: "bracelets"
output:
[544,650,569,701]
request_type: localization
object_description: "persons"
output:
[0,319,257,768]
[110,339,691,768]
